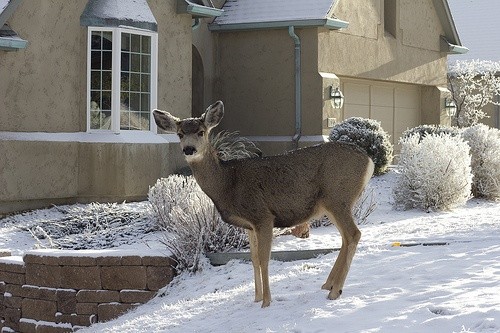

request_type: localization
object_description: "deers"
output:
[153,100,376,308]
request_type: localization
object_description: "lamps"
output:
[329,84,344,109]
[444,95,458,117]
